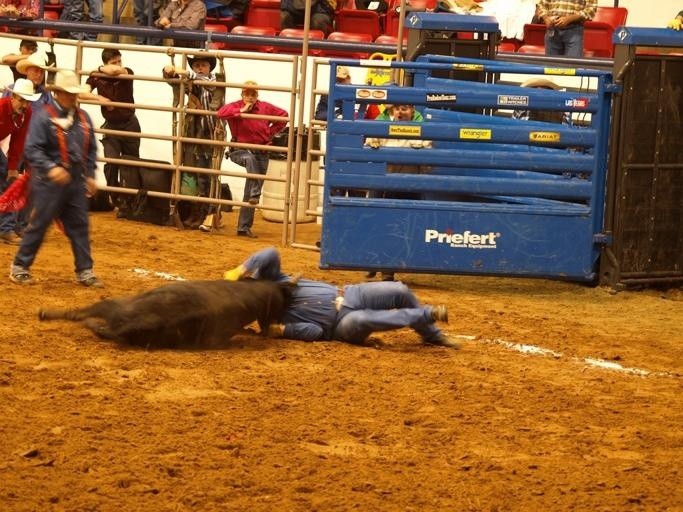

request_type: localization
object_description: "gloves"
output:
[268,323,286,339]
[223,265,245,282]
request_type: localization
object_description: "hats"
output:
[335,65,351,80]
[14,50,63,77]
[1,78,44,104]
[18,38,39,48]
[183,48,218,74]
[41,68,92,98]
[240,79,260,95]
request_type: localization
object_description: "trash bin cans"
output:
[259,128,321,223]
[43,4,64,37]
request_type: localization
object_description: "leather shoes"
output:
[422,330,464,351]
[428,300,451,325]
[235,226,260,239]
[246,195,261,206]
[114,207,128,221]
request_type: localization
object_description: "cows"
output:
[115,154,232,230]
[37,271,305,350]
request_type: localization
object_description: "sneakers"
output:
[364,269,395,282]
[0,228,25,247]
[76,267,106,290]
[8,265,38,286]
[195,222,212,232]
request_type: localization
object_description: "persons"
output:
[536,0,598,57]
[1,0,427,287]
[224,246,462,348]
[667,10,683,32]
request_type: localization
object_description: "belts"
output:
[332,285,346,317]
